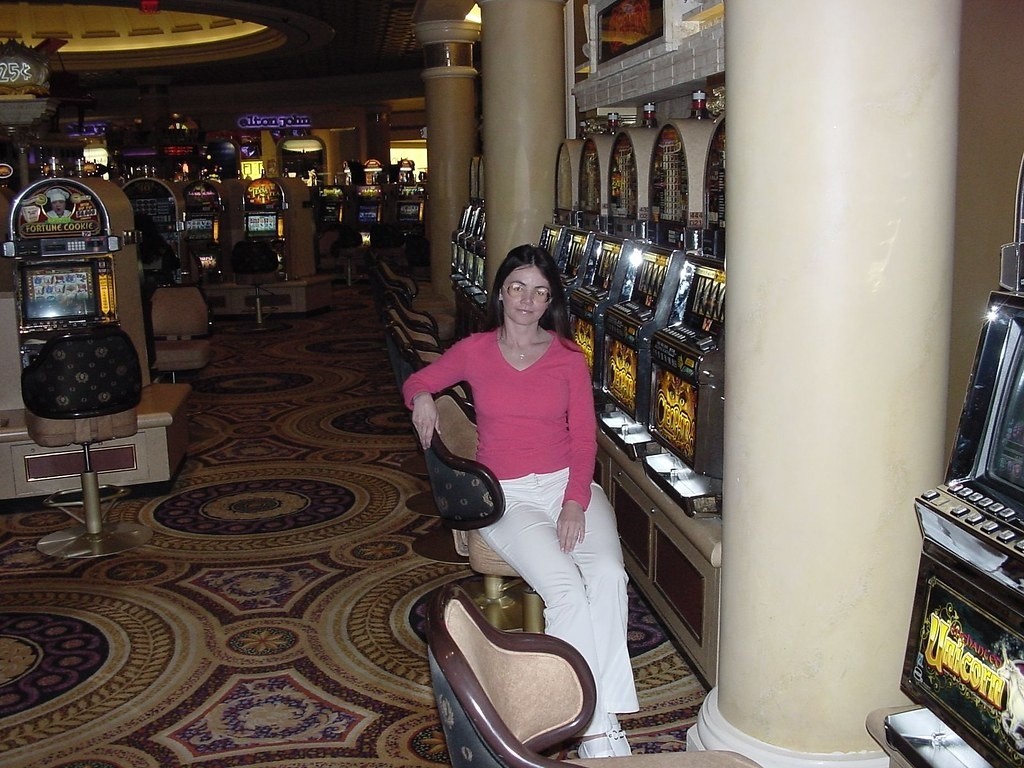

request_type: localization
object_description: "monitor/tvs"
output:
[246,212,279,238]
[20,261,102,326]
[984,330,1024,503]
[186,216,215,241]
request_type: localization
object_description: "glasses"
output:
[503,281,554,304]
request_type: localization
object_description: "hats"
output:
[45,189,70,204]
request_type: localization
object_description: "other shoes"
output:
[577,737,617,759]
[604,724,632,757]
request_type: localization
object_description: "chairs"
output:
[149,286,211,384]
[367,248,475,564]
[20,322,153,559]
[322,220,430,297]
[426,582,763,768]
[410,388,545,634]
[225,240,293,335]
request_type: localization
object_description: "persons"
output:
[400,244,641,761]
[45,188,71,218]
